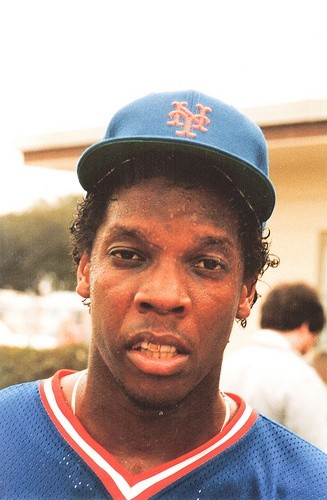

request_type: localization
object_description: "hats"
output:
[77,90,276,232]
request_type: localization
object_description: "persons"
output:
[219,282,326,456]
[0,88,326,500]
